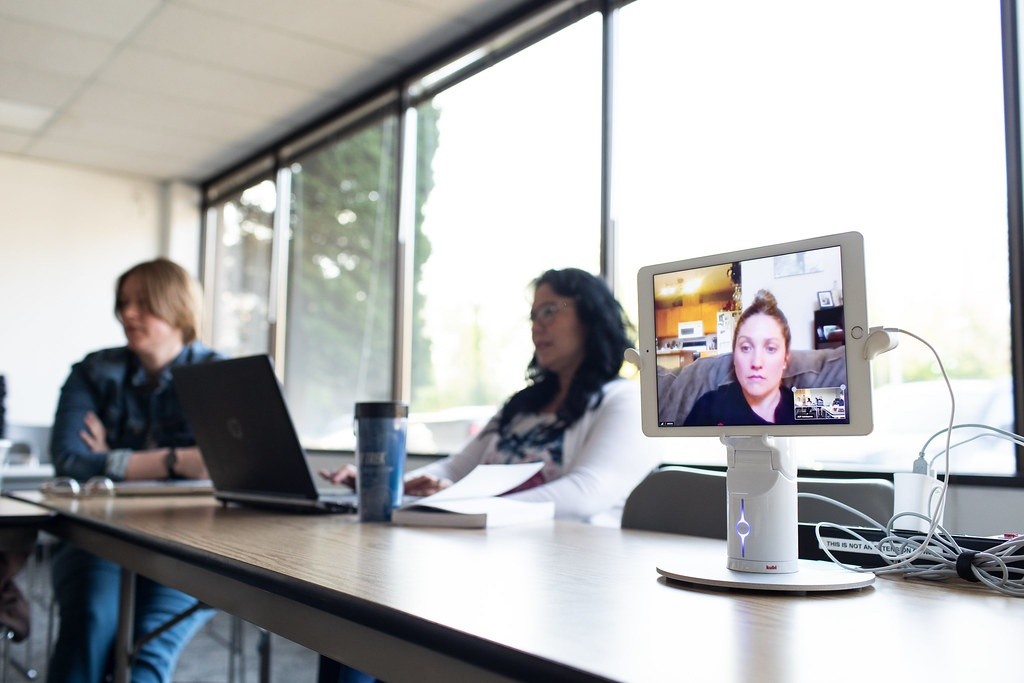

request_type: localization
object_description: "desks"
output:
[10,489,1024,683]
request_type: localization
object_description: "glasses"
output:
[43,475,116,499]
[526,302,580,328]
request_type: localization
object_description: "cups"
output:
[353,402,409,520]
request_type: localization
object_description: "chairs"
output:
[620,465,895,539]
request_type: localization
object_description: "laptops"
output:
[172,355,428,514]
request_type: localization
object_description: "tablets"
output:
[635,231,873,438]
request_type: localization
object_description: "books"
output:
[390,461,555,529]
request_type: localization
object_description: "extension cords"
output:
[796,523,1024,580]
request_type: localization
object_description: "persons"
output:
[49,253,229,682]
[684,289,847,425]
[318,265,665,528]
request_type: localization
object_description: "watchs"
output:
[163,447,179,475]
